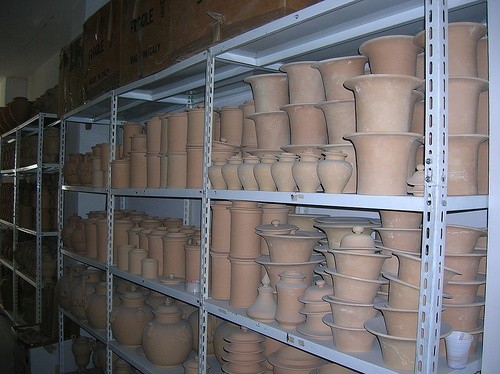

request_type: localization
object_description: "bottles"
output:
[1,84,62,321]
[62,34,500,374]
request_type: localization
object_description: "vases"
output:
[0,83,68,337]
[54,21,491,374]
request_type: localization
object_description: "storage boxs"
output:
[57,0,325,113]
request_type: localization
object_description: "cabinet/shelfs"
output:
[60,0,500,374]
